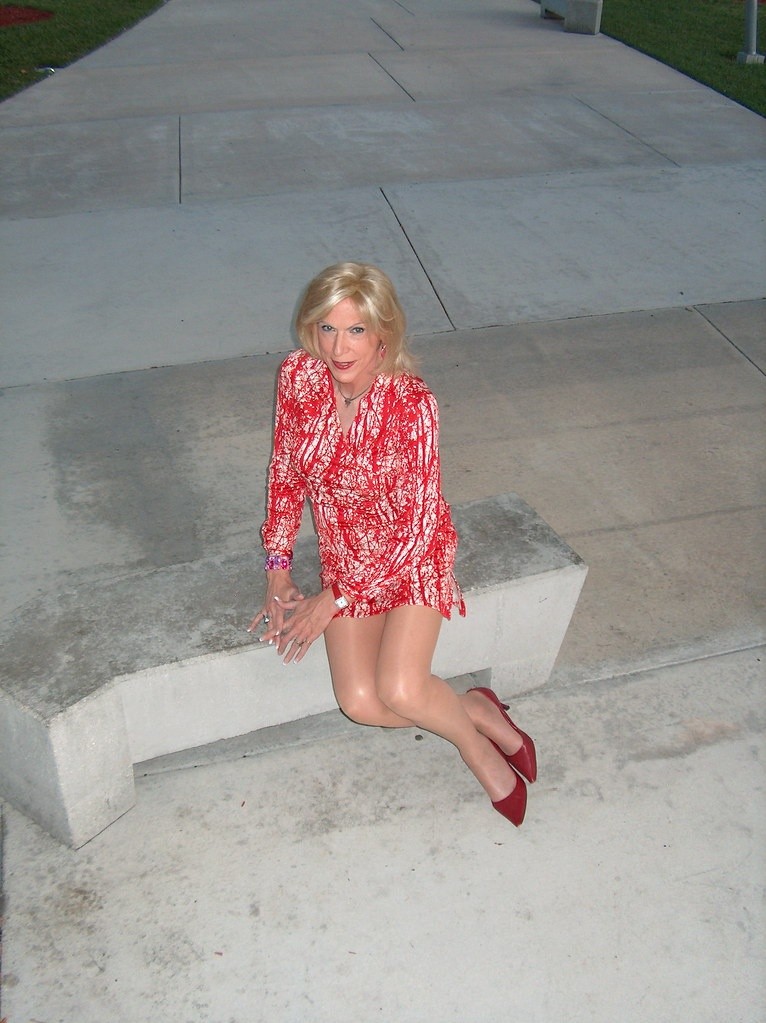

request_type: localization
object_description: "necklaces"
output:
[338,384,370,406]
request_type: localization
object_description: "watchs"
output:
[331,583,349,609]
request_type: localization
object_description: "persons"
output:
[248,262,538,829]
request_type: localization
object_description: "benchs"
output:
[0,496,587,850]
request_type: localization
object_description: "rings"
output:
[305,640,312,644]
[296,641,301,645]
[264,618,269,623]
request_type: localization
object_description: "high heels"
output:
[487,737,526,827]
[467,687,537,783]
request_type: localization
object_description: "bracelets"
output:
[264,557,292,572]
